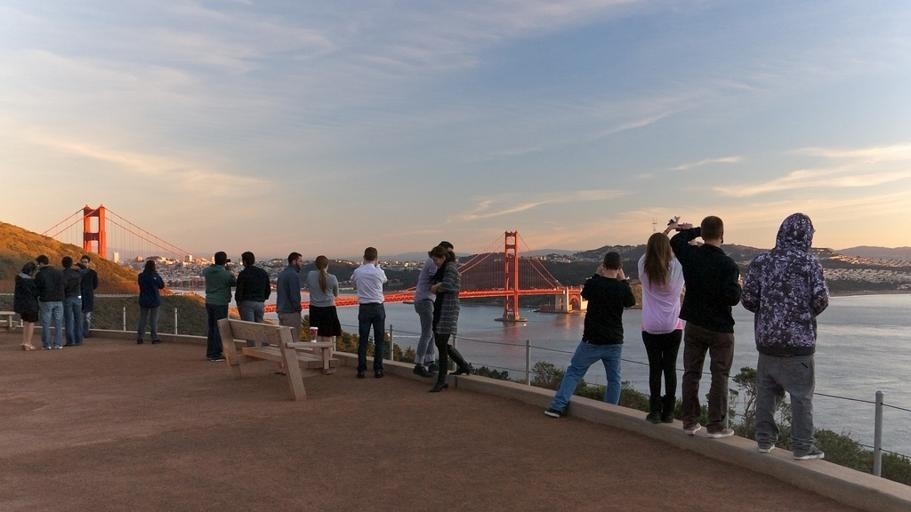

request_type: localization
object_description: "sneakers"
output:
[21,342,83,351]
[544,409,560,418]
[152,339,161,343]
[137,340,143,343]
[355,360,473,392]
[647,410,819,460]
[210,354,225,363]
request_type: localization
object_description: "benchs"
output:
[218,316,333,402]
[0,311,17,327]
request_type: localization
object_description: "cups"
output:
[310,327,318,343]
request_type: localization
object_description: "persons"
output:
[638,215,686,424]
[13,253,98,351]
[670,216,743,439]
[307,255,341,376]
[202,251,271,362]
[741,212,830,460]
[412,241,454,377]
[543,252,636,419]
[428,245,470,392]
[276,252,303,375]
[137,260,165,344]
[349,247,389,378]
